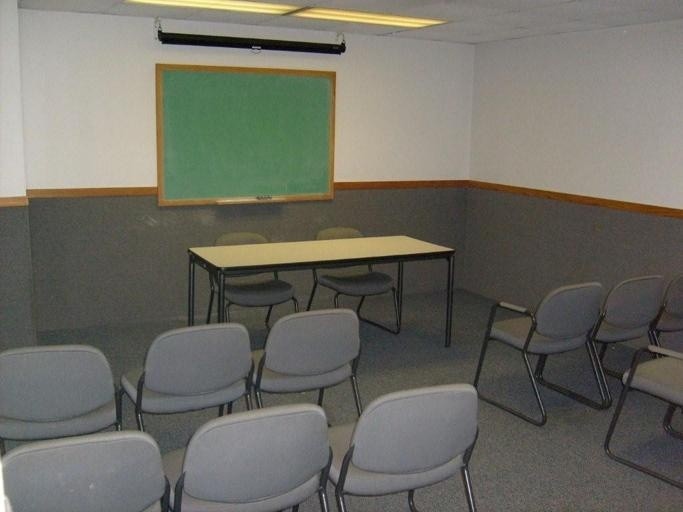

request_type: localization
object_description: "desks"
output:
[187,235,456,347]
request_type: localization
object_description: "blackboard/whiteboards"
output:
[153,60,339,210]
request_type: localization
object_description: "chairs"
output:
[119,322,254,433]
[227,307,364,428]
[206,232,299,324]
[0,345,122,455]
[472,282,606,426]
[167,402,330,512]
[1,430,170,511]
[603,342,683,489]
[651,273,683,360]
[306,226,400,335]
[590,274,660,411]
[325,384,480,512]
